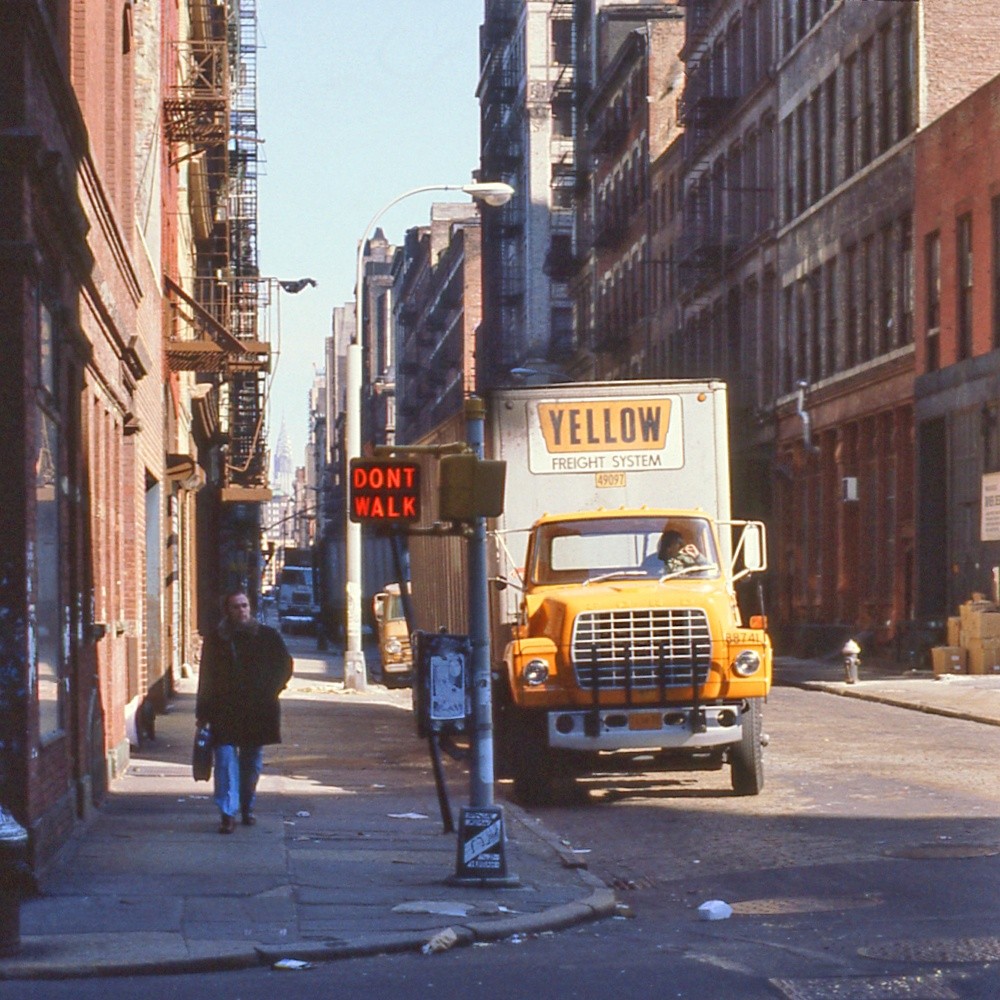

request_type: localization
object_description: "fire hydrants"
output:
[841,638,862,684]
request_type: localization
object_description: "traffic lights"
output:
[349,457,423,525]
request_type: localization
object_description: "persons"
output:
[313,597,332,649]
[659,531,718,578]
[195,587,294,833]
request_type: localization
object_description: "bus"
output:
[373,580,415,681]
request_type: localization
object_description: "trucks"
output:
[273,567,319,623]
[392,377,776,803]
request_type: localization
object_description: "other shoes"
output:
[242,808,255,823]
[221,814,235,834]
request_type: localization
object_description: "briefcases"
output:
[192,729,213,782]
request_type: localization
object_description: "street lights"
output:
[345,181,515,690]
